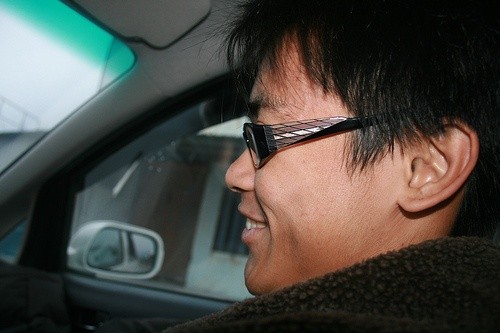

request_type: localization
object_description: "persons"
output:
[162,0,500,333]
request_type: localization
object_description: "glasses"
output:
[243,110,433,168]
